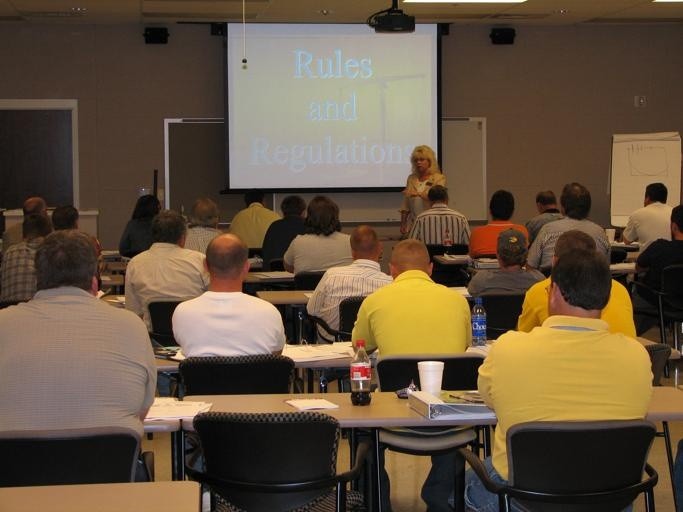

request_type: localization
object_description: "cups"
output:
[417,360,445,395]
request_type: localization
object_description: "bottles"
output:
[470,296,486,346]
[442,228,453,254]
[349,340,371,407]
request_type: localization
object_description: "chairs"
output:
[467,293,526,342]
[645,344,670,386]
[454,418,658,512]
[192,410,363,512]
[314,297,372,394]
[148,298,192,347]
[291,270,326,338]
[626,263,683,378]
[171,354,302,482]
[369,355,486,512]
[0,427,154,484]
[269,259,284,273]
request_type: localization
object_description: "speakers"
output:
[492,21,522,44]
[144,16,180,42]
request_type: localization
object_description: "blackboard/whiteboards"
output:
[0,98,79,212]
[164,114,488,225]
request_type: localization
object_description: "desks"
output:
[257,291,315,345]
[155,343,358,395]
[98,294,124,308]
[100,275,124,294]
[433,255,471,288]
[140,396,181,483]
[103,262,129,274]
[183,386,682,511]
[241,270,295,296]
[101,250,121,261]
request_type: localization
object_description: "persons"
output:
[445,249,654,511]
[526,190,566,238]
[0,212,53,302]
[1,229,158,430]
[284,195,351,279]
[125,210,210,331]
[408,186,470,245]
[400,145,447,240]
[468,228,540,295]
[630,204,683,336]
[182,199,223,253]
[307,227,392,344]
[0,197,46,256]
[53,206,79,229]
[352,239,472,507]
[119,194,162,258]
[262,196,309,272]
[172,233,285,356]
[469,190,530,256]
[527,182,611,277]
[229,190,282,248]
[518,230,636,338]
[623,183,673,250]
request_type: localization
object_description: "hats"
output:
[496,228,527,258]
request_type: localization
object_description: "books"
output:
[254,270,295,279]
[407,389,497,421]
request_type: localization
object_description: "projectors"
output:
[366,1,415,34]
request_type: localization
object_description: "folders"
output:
[407,391,497,419]
